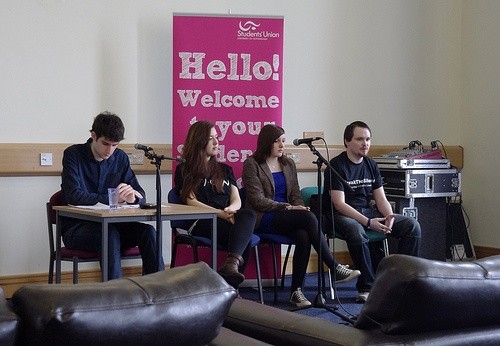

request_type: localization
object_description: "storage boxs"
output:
[379,169,462,199]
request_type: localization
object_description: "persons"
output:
[61,113,165,282]
[173,120,256,289]
[242,124,362,307]
[323,121,422,303]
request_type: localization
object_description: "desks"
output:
[51,204,220,283]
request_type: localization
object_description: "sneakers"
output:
[335,263,362,284]
[289,287,312,308]
[355,291,370,303]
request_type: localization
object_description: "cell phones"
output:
[140,204,156,209]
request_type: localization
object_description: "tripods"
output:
[290,142,357,325]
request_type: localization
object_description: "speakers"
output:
[371,196,476,262]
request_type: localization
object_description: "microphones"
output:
[293,137,321,146]
[134,144,153,150]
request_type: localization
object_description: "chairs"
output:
[45,189,141,284]
[166,187,265,306]
[0,261,269,346]
[300,186,389,300]
[221,253,500,346]
[240,187,329,304]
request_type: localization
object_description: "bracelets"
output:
[367,218,371,230]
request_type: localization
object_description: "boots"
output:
[218,252,246,286]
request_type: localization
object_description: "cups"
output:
[108,188,119,210]
